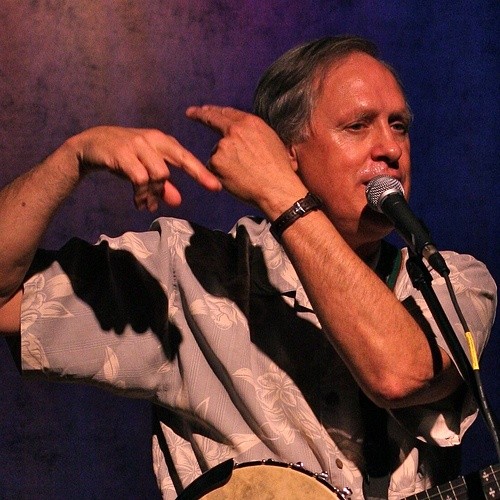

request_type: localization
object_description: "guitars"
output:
[168,458,499,499]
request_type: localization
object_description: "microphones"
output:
[366,177,451,277]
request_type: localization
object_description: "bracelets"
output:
[271,191,325,243]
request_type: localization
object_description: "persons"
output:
[1,37,499,499]
